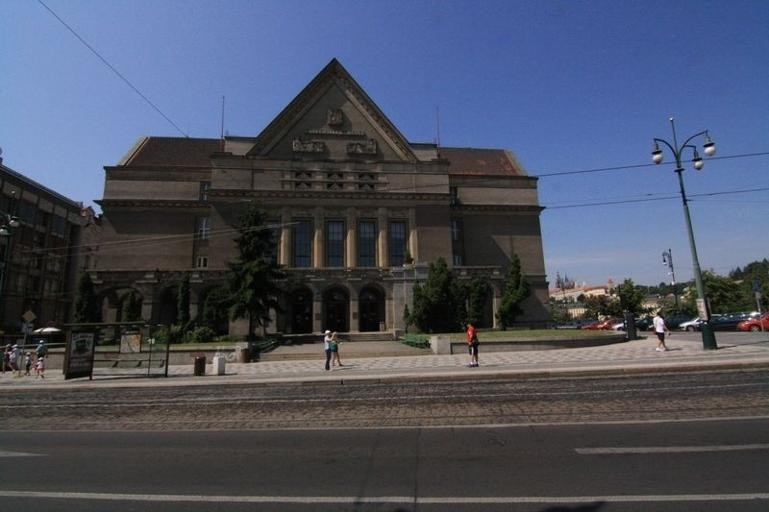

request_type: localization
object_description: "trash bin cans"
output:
[194,357,206,376]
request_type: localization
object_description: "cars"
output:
[554,311,769,331]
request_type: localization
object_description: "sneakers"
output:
[467,362,480,367]
[656,346,667,353]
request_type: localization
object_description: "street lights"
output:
[0,191,21,347]
[752,291,764,331]
[651,117,718,350]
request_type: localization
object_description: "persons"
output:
[652,311,670,352]
[2,340,49,379]
[464,320,479,366]
[323,329,343,370]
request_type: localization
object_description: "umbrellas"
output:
[31,325,62,343]
